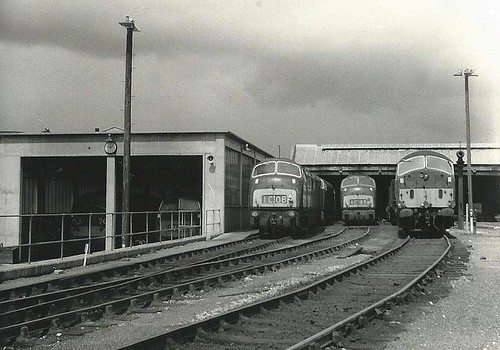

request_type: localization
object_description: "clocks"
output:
[104,141,118,154]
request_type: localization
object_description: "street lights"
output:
[118,15,142,250]
[453,67,480,235]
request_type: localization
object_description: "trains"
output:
[380,151,457,238]
[247,157,336,242]
[340,175,377,227]
[69,191,158,244]
[156,192,202,239]
